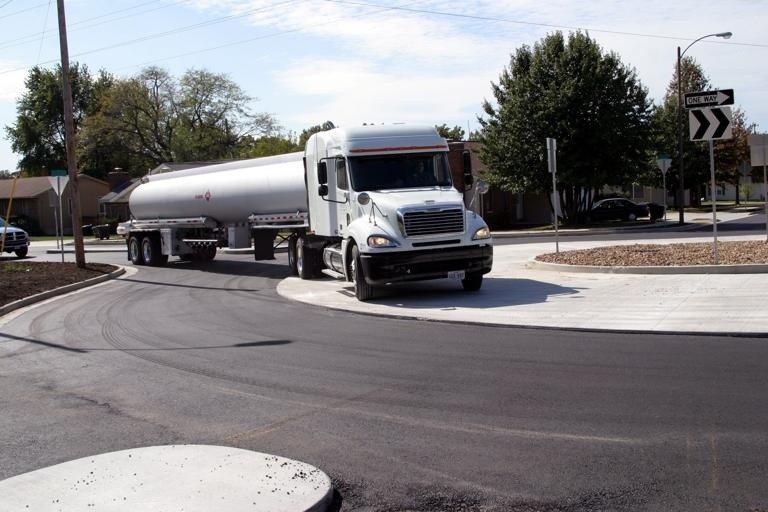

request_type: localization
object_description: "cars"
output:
[589,196,650,222]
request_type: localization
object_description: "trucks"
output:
[116,119,494,305]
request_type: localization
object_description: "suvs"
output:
[0,218,32,259]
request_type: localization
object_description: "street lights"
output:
[675,28,736,227]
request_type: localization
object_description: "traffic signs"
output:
[684,85,739,110]
